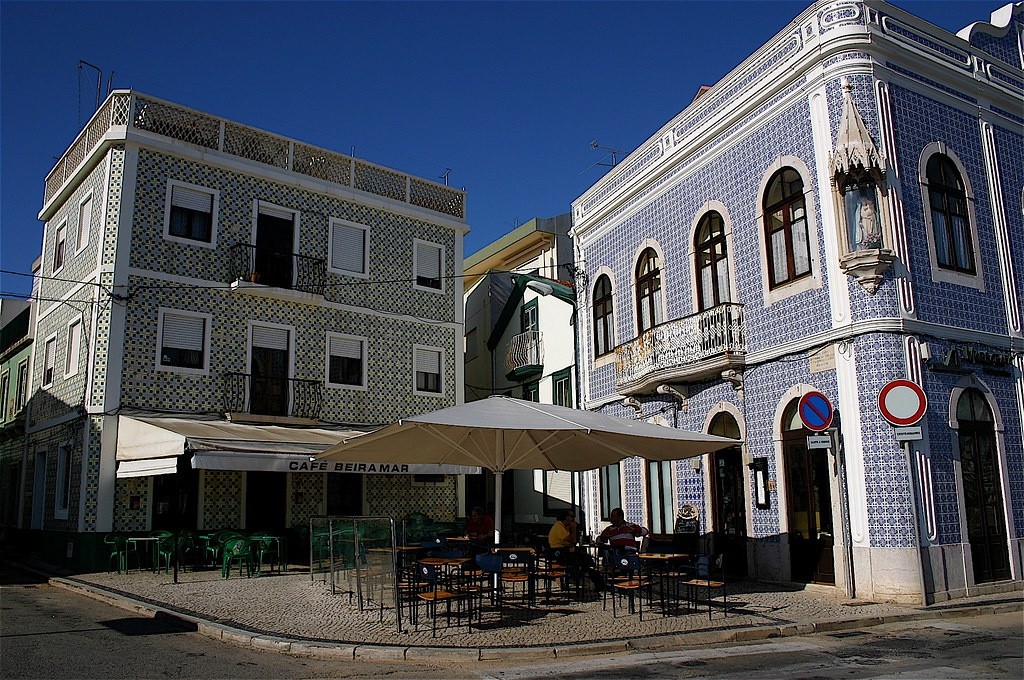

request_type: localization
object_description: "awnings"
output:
[116,414,482,477]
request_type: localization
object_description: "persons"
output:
[462,506,495,559]
[596,508,649,577]
[548,510,604,590]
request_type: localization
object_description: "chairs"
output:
[102,527,281,581]
[326,531,728,637]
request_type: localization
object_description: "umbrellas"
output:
[307,392,745,594]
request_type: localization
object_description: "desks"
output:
[123,536,160,576]
[627,552,692,615]
[365,545,422,599]
[580,542,613,591]
[248,536,288,574]
[490,546,534,604]
[419,556,474,626]
